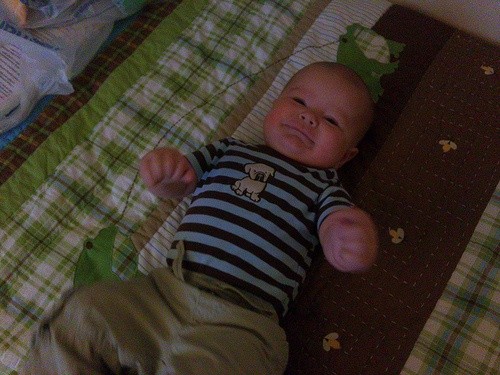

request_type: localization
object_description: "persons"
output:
[33,62,378,375]
